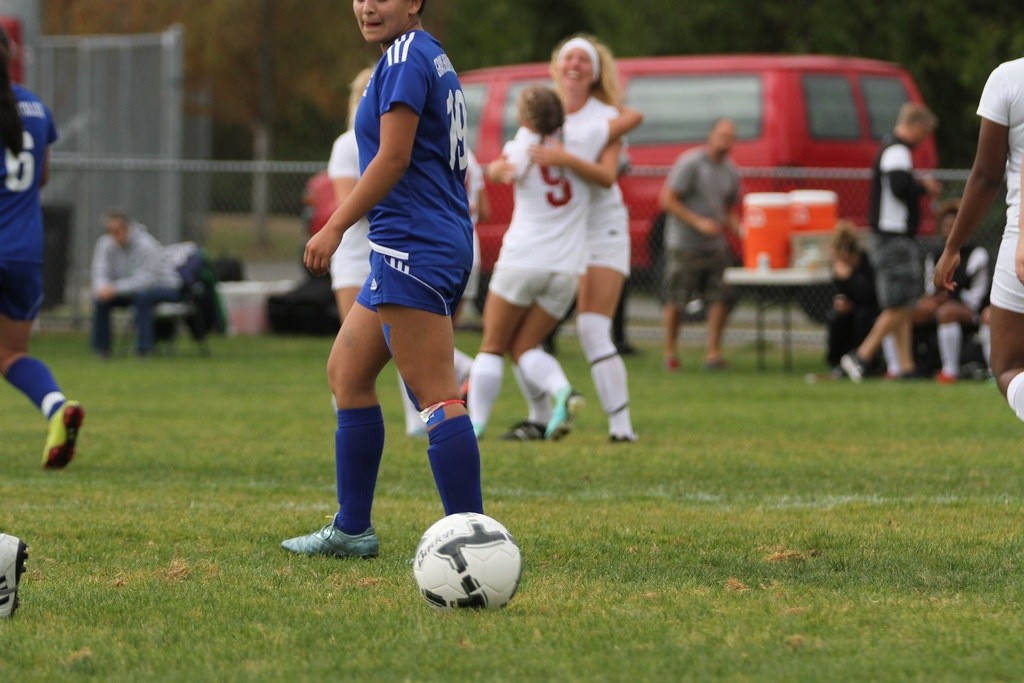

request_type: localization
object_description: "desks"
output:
[722,262,836,368]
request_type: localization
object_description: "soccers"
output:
[412,510,523,614]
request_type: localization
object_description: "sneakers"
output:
[545,388,586,442]
[281,516,379,559]
[454,358,476,403]
[498,420,548,441]
[41,400,84,470]
[0,535,30,619]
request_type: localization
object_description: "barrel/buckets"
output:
[742,188,839,268]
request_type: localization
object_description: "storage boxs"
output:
[217,281,298,336]
[740,190,840,272]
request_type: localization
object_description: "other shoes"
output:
[936,370,958,382]
[704,357,736,374]
[664,357,682,372]
[841,353,866,382]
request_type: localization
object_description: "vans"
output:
[301,56,938,322]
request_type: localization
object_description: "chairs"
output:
[116,238,214,358]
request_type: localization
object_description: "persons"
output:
[89,214,181,359]
[328,34,1024,441]
[0,530,30,620]
[0,26,85,470]
[281,0,485,559]
